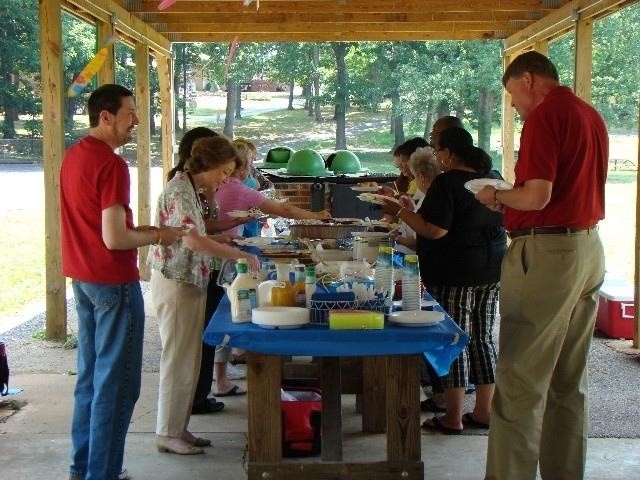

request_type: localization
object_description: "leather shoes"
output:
[157,435,202,455]
[184,430,212,446]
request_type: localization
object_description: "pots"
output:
[349,231,398,263]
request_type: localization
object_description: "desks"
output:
[204,217,461,480]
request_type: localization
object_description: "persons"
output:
[60,84,187,480]
[474,51,608,480]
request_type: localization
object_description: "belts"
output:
[505,224,596,240]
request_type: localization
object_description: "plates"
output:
[224,210,250,217]
[386,309,446,329]
[233,235,277,247]
[250,305,311,329]
[357,193,400,206]
[349,185,383,192]
[180,222,196,234]
[463,177,514,196]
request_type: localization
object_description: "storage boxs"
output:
[280,387,324,459]
[596,285,635,340]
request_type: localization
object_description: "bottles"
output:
[228,263,259,324]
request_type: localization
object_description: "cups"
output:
[374,246,395,301]
[275,264,291,282]
[293,265,317,308]
[401,255,422,311]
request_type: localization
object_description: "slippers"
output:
[463,412,490,428]
[422,415,463,434]
[212,385,247,397]
[419,396,448,414]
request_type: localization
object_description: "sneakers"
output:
[67,466,128,480]
[192,402,224,414]
[205,398,218,402]
